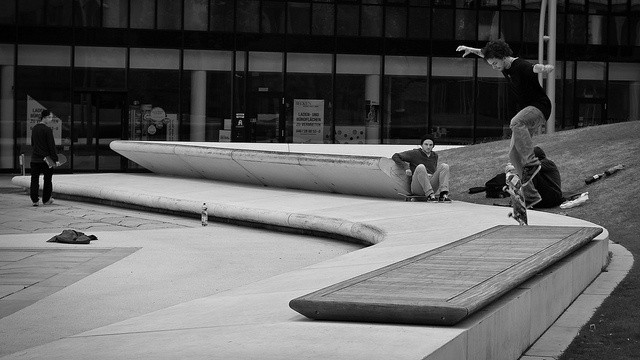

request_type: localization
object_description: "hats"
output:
[421,134,434,148]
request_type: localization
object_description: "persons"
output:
[527,146,562,208]
[392,134,452,203]
[29,109,61,207]
[455,38,554,211]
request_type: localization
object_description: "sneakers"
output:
[521,164,541,186]
[440,196,451,202]
[526,198,542,208]
[427,196,439,202]
[32,201,39,206]
[44,198,55,205]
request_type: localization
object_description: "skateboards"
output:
[502,172,527,225]
[398,193,451,201]
[44,153,67,168]
